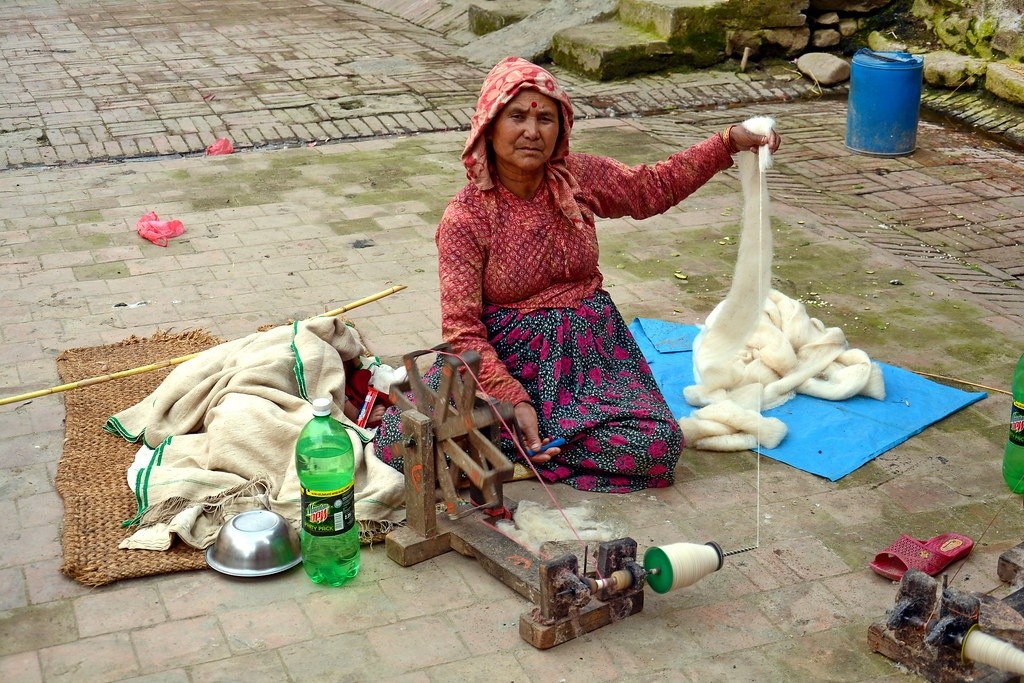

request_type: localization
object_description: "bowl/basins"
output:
[205,510,303,577]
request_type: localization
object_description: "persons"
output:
[345,56,780,494]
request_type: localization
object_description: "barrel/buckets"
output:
[843,48,925,156]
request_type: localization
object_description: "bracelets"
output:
[723,124,739,154]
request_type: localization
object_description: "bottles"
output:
[1001,352,1024,495]
[295,398,361,588]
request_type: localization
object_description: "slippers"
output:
[870,531,974,581]
